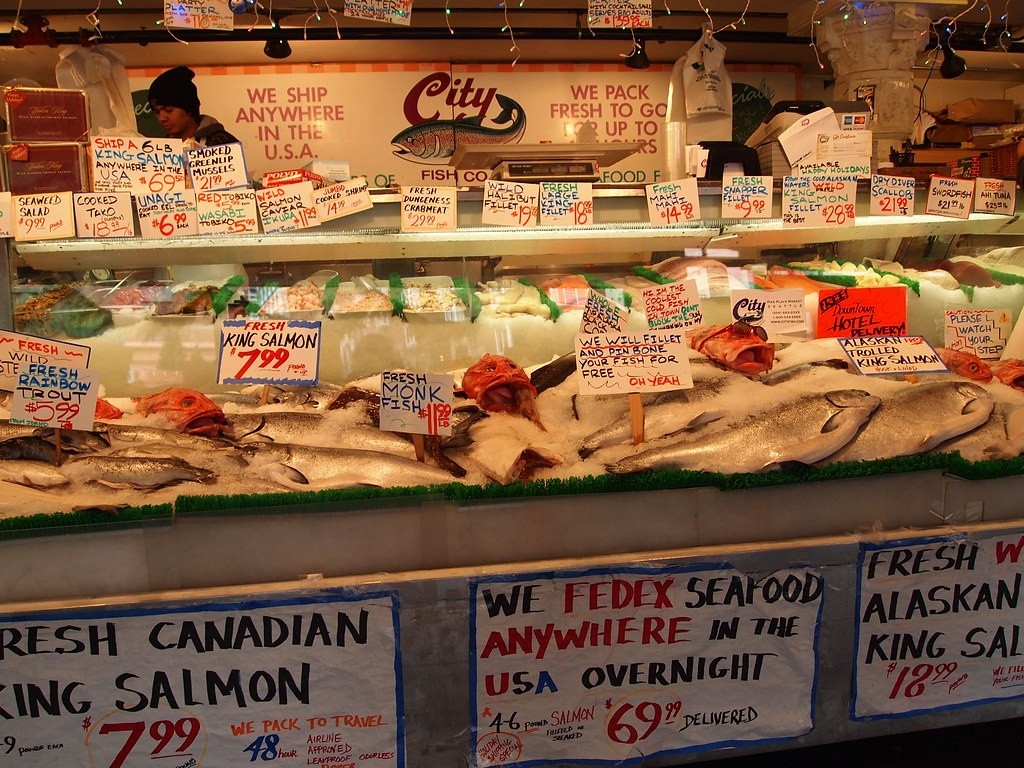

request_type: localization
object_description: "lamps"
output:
[263,17,294,61]
[932,28,968,80]
[623,38,651,71]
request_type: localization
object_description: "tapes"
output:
[878,162,894,169]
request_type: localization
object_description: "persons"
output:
[147,66,239,146]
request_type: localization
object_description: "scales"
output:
[447,142,647,182]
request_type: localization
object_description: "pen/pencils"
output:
[890,146,912,155]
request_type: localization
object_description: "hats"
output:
[148,66,199,114]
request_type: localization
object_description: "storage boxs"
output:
[4,86,94,147]
[0,140,83,197]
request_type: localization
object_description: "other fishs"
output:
[0,314,1024,492]
